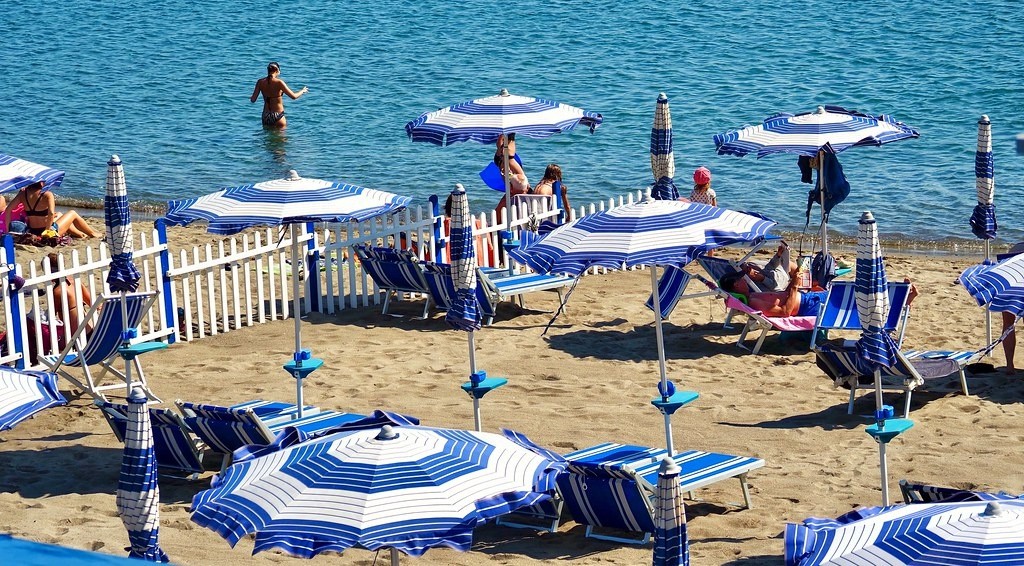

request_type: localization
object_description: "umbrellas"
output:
[958,253,1024,323]
[713,105,919,253]
[165,170,412,364]
[855,211,899,418]
[783,490,1024,566]
[116,387,169,564]
[404,87,604,220]
[969,114,997,242]
[190,411,570,558]
[0,154,65,194]
[105,154,141,347]
[509,193,778,401]
[445,183,485,373]
[652,457,690,566]
[650,92,679,202]
[0,365,68,432]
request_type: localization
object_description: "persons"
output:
[1002,242,1024,372]
[493,133,529,226]
[719,246,918,317]
[4,181,102,238]
[535,164,571,223]
[41,253,99,354]
[250,62,309,128]
[690,167,717,257]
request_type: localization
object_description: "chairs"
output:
[900,480,963,505]
[352,193,580,327]
[497,442,766,545]
[35,288,366,485]
[644,257,981,419]
